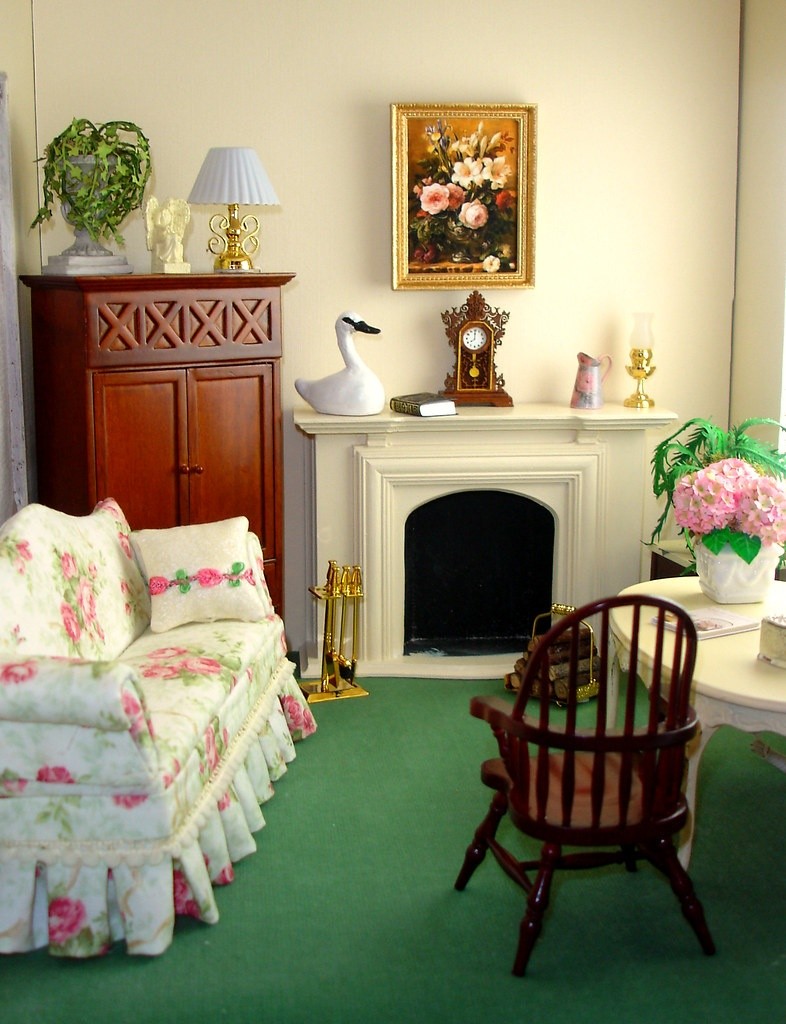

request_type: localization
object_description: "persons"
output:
[152,197,183,263]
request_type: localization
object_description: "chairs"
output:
[458,594,718,979]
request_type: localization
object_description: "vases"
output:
[691,536,784,604]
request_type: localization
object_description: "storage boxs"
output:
[758,613,786,669]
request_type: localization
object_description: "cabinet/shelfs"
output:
[647,539,786,582]
[17,271,298,620]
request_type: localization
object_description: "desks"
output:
[605,577,786,872]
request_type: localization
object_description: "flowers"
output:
[639,414,786,576]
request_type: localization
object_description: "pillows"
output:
[136,515,268,633]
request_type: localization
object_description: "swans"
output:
[295,311,385,415]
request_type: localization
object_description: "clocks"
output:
[438,290,514,407]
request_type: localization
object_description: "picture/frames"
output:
[649,606,760,641]
[389,101,537,290]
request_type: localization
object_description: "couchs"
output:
[0,498,319,960]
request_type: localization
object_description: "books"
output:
[390,392,459,416]
[652,606,760,641]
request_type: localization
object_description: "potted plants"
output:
[28,116,152,255]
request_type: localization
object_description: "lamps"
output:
[187,147,282,272]
